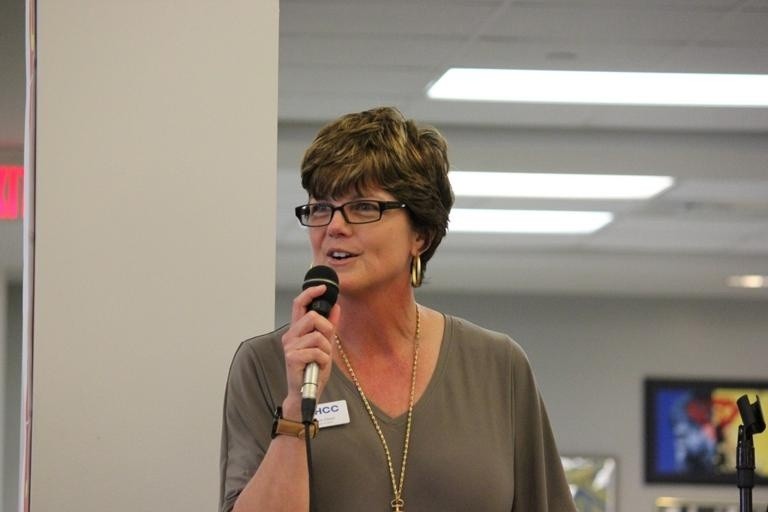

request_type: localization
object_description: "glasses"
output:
[295,200,407,228]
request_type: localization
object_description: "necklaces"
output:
[332,304,420,512]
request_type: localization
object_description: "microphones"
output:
[302,265,339,427]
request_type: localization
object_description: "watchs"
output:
[270,405,318,439]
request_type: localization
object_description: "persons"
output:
[173,64,574,512]
